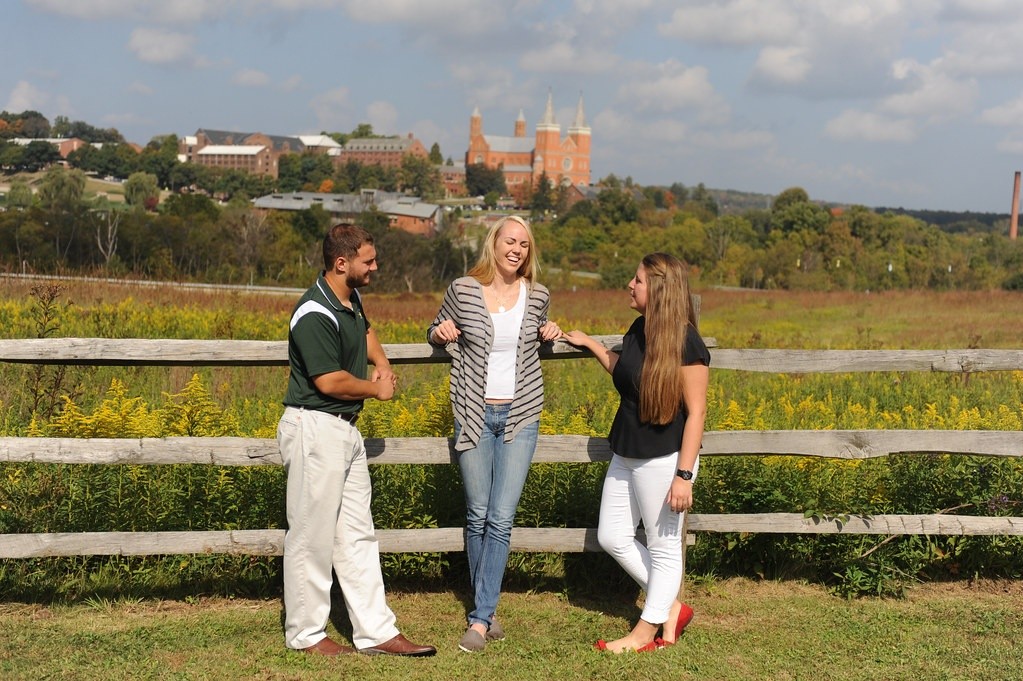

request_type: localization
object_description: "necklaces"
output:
[489,287,514,314]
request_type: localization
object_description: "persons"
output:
[426,215,562,651]
[277,223,435,654]
[562,252,711,656]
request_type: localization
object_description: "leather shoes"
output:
[359,630,437,655]
[295,636,353,655]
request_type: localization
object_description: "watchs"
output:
[676,469,693,480]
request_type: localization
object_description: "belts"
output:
[331,411,360,426]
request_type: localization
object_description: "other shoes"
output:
[657,602,692,648]
[485,617,505,641]
[593,639,655,655]
[458,628,485,653]
